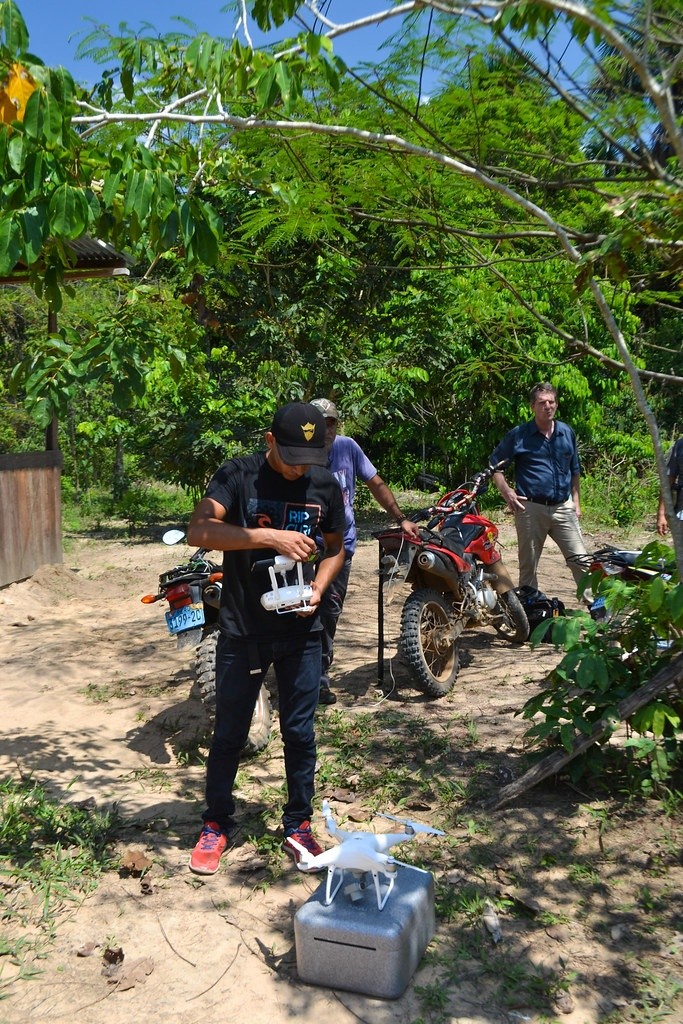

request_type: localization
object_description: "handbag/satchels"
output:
[501,585,566,643]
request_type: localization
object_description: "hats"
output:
[271,397,330,467]
[309,398,338,420]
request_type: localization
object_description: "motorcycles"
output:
[370,456,531,699]
[565,510,683,657]
[140,529,276,759]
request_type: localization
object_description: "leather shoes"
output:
[319,691,337,704]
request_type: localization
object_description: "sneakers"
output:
[188,822,231,874]
[283,821,328,873]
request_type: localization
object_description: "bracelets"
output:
[396,514,407,526]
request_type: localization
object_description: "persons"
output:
[490,382,607,622]
[187,403,348,875]
[657,436,683,537]
[310,398,419,704]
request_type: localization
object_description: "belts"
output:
[524,496,569,505]
